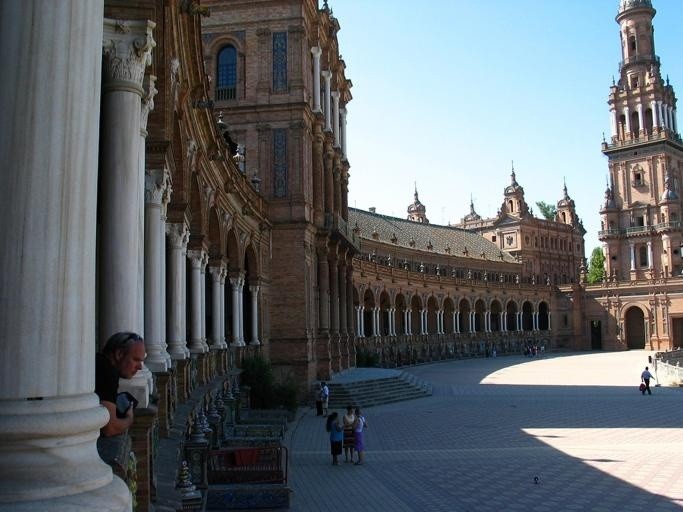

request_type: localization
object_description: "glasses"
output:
[122,332,140,344]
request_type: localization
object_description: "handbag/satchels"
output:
[639,384,645,391]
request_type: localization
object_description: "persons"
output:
[320,380,329,418]
[525,342,545,359]
[640,367,654,395]
[315,382,323,416]
[351,407,367,465]
[491,343,497,359]
[484,339,490,358]
[96,331,147,438]
[341,404,355,463]
[325,411,343,466]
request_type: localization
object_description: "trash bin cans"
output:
[317,401,323,415]
